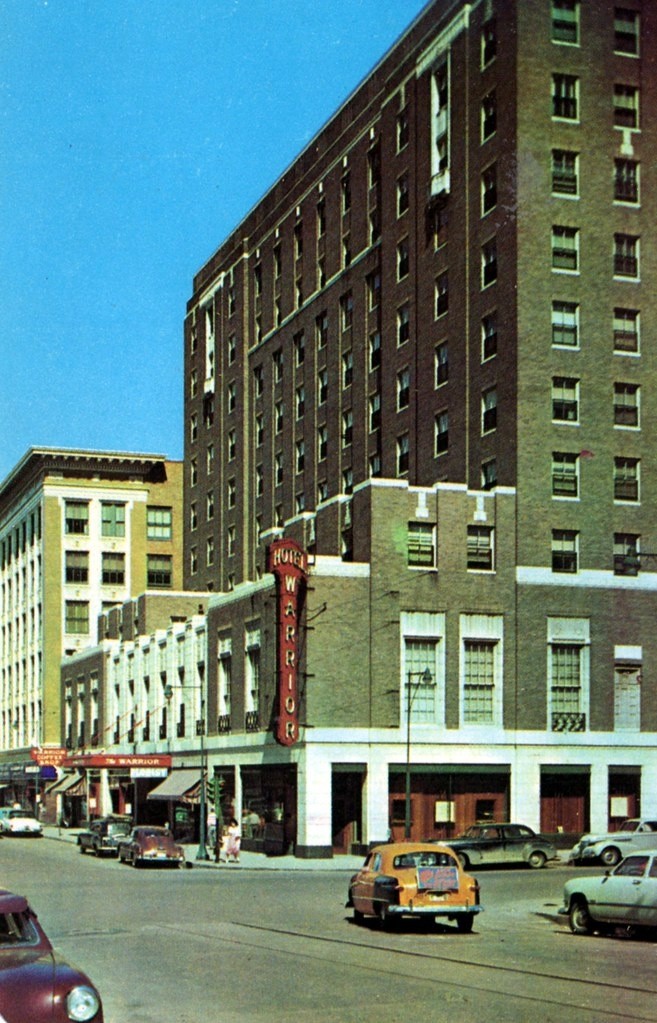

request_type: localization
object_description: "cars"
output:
[0,886,107,1023]
[0,806,43,835]
[343,841,485,933]
[116,824,186,867]
[568,817,657,867]
[76,818,134,857]
[425,822,558,871]
[558,849,657,934]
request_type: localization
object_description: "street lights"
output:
[405,667,433,839]
[164,682,210,860]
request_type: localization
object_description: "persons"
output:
[207,808,218,850]
[224,818,241,864]
[247,809,261,836]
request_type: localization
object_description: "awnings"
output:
[146,769,207,805]
[44,775,88,796]
